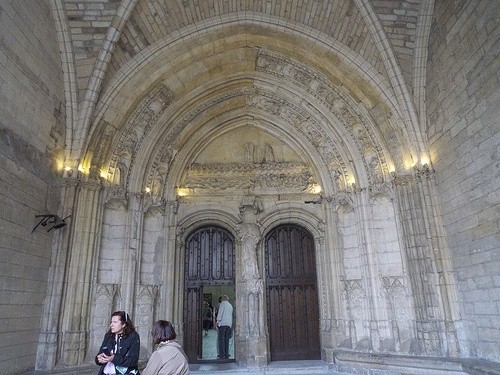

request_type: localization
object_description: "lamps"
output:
[32,214,71,233]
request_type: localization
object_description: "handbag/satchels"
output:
[206,312,212,318]
[116,366,138,375]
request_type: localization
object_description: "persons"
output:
[141,320,192,375]
[215,295,233,360]
[202,302,213,335]
[213,297,231,358]
[94,310,141,375]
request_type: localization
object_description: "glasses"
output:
[117,311,128,322]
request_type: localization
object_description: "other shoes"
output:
[205,333,208,335]
[219,356,229,359]
